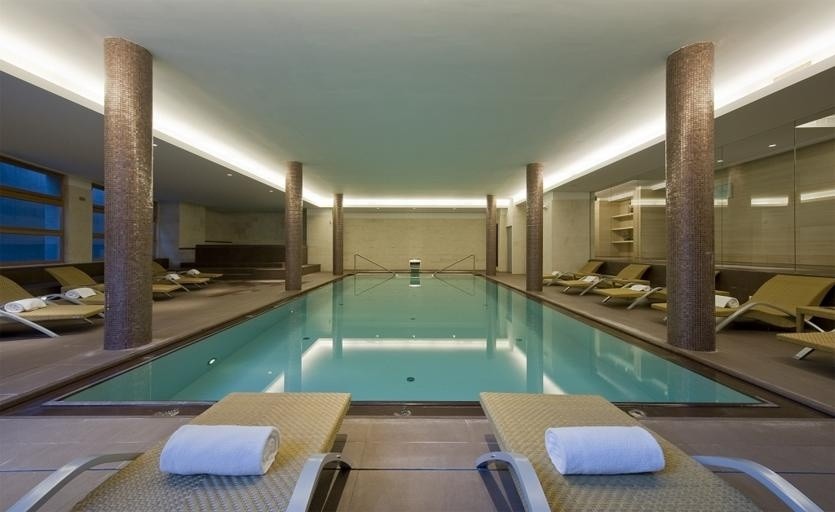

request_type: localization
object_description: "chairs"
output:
[475,392,825,512]
[0,262,223,338]
[543,261,835,360]
[5,392,353,512]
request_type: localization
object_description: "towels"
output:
[159,425,282,476]
[544,426,665,476]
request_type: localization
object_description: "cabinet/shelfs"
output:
[611,213,633,243]
